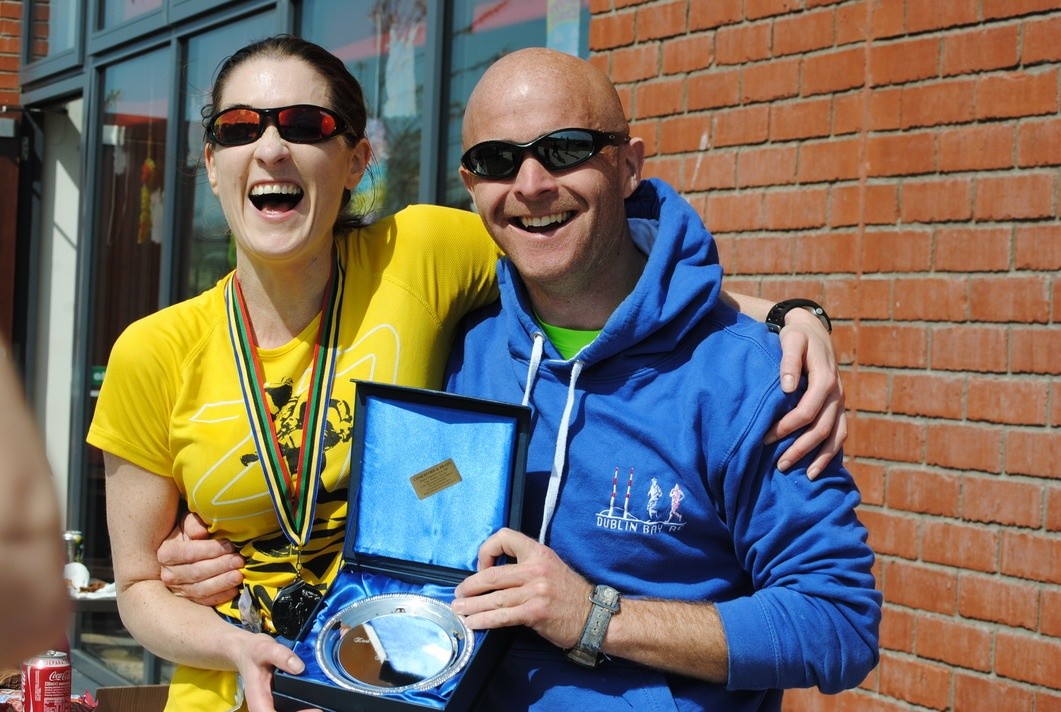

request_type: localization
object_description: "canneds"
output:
[29,650,71,711]
[21,656,33,712]
[64,530,87,564]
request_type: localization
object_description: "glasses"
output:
[460,127,632,180]
[204,103,361,145]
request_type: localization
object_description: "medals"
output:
[268,579,325,641]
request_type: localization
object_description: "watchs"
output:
[762,298,832,339]
[560,581,621,673]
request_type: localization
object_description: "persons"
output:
[153,43,883,711]
[1,334,73,686]
[79,33,850,711]
